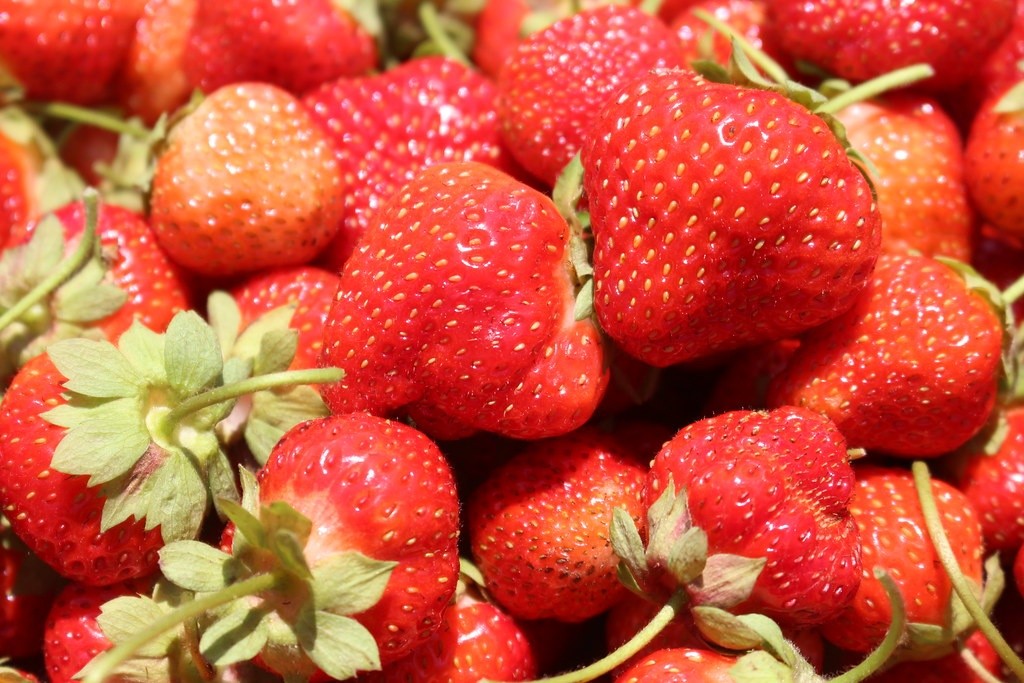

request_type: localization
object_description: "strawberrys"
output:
[1,0,1024,683]
